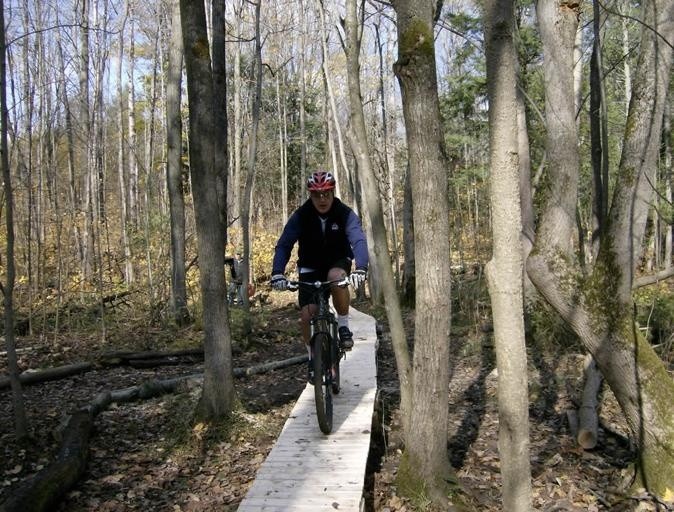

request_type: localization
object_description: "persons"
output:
[266,170,371,385]
[224,251,244,306]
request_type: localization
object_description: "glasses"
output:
[311,190,332,198]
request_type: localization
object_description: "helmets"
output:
[307,171,336,190]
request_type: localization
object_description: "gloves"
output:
[350,270,367,290]
[271,274,288,291]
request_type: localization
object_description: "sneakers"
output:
[339,328,354,347]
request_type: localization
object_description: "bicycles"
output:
[270,276,361,434]
